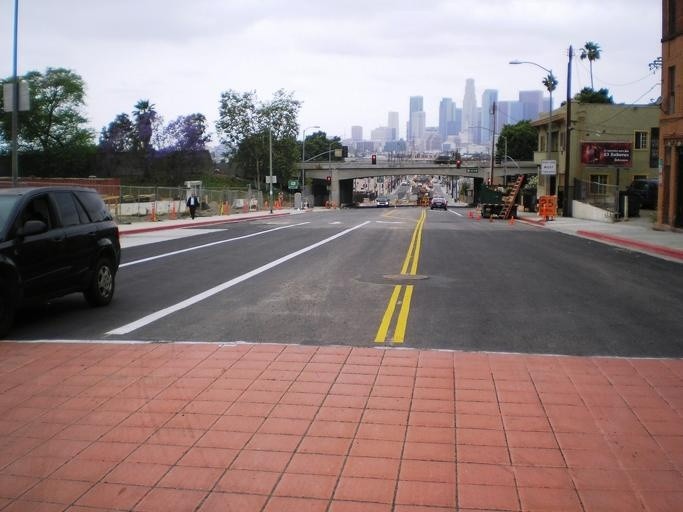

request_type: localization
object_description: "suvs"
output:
[376,196,390,208]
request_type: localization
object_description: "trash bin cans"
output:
[304,201,309,209]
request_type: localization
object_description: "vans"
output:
[625,178,659,210]
[433,155,452,164]
[0,184,121,308]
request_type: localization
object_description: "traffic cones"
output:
[469,212,481,221]
[510,215,514,224]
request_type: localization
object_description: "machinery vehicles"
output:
[476,183,518,219]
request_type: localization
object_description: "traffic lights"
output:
[456,160,461,168]
[372,155,376,164]
[327,177,331,185]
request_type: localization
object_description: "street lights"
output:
[302,125,320,191]
[328,140,343,208]
[509,60,553,222]
[468,126,507,188]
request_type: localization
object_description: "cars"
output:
[430,197,448,211]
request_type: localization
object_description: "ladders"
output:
[498,175,524,219]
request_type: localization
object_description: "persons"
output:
[186,191,199,220]
